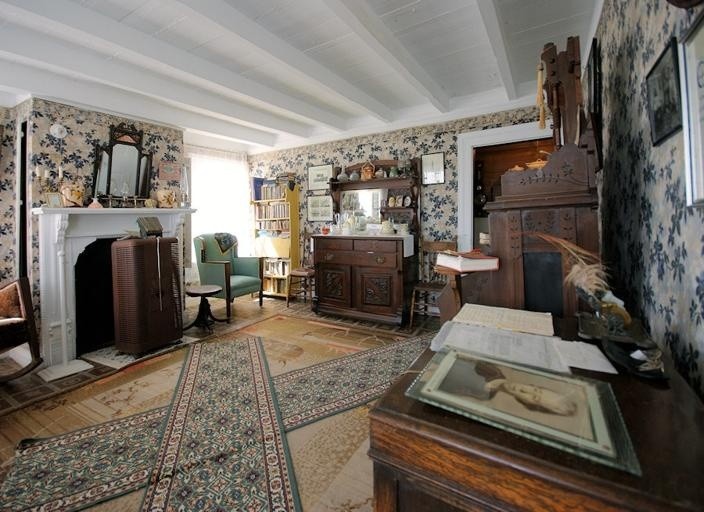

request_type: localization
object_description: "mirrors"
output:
[92,121,153,204]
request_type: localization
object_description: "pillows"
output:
[0,284,21,319]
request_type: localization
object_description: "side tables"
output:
[183,284,228,335]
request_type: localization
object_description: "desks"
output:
[366,302,703,511]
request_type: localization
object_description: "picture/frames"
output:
[421,151,445,185]
[404,345,643,478]
[676,10,704,207]
[646,37,681,147]
[45,192,64,208]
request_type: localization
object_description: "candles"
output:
[94,170,99,198]
[36,166,41,176]
[59,166,63,180]
[45,170,50,179]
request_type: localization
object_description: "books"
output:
[435,249,499,273]
[250,171,298,295]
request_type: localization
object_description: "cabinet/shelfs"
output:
[310,159,420,327]
[250,176,299,300]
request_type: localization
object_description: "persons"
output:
[437,356,578,423]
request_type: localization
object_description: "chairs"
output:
[0,277,44,383]
[287,232,315,307]
[193,233,262,318]
[409,241,457,328]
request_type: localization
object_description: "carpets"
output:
[138,336,305,512]
[0,331,444,512]
